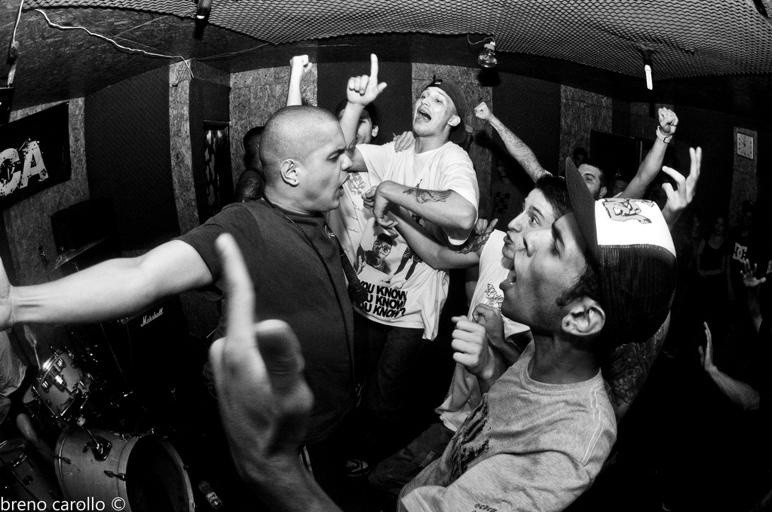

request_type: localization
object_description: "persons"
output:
[612,177,628,195]
[339,51,480,395]
[602,146,702,423]
[726,204,755,300]
[384,245,422,289]
[288,54,379,270]
[358,174,573,487]
[364,233,391,274]
[696,259,772,417]
[474,102,678,199]
[696,215,726,278]
[208,158,676,512]
[236,126,267,204]
[0,105,363,486]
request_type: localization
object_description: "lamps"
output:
[466,35,498,69]
[643,51,653,90]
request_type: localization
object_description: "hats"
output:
[426,79,466,143]
[565,157,676,344]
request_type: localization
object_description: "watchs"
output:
[656,126,673,144]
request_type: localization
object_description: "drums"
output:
[0,439,62,512]
[32,351,83,417]
[54,426,196,512]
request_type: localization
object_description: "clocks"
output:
[736,133,754,160]
